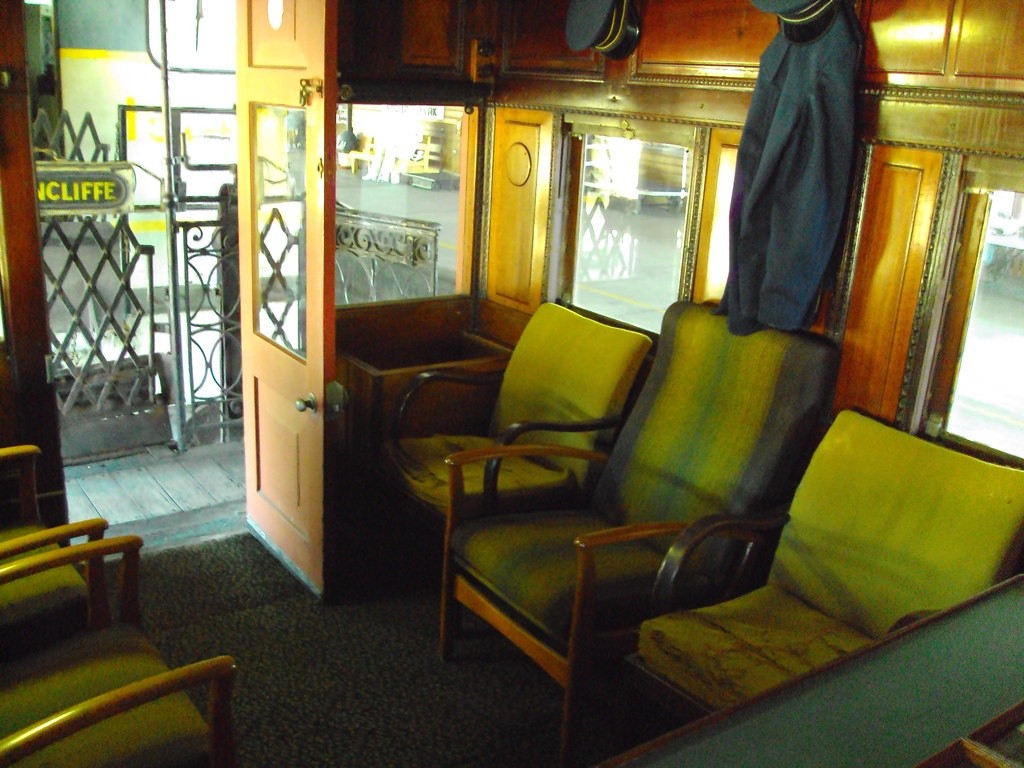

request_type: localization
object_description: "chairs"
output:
[0,535,236,768]
[619,400,1024,741]
[372,297,660,643]
[437,301,839,768]
[0,444,111,655]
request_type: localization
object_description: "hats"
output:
[751,0,839,45]
[565,0,640,60]
